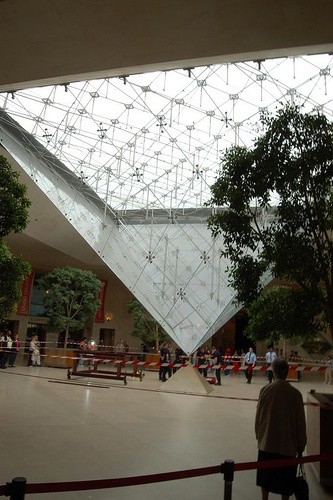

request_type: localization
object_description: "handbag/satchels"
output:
[294,458,309,500]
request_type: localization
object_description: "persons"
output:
[265,345,278,384]
[79,337,106,367]
[114,338,129,367]
[141,343,148,362]
[0,330,12,369]
[288,350,302,379]
[28,336,41,367]
[173,344,211,378]
[211,347,224,385]
[243,348,256,383]
[159,342,172,381]
[254,358,307,500]
[324,355,333,384]
[224,348,245,376]
[8,334,20,368]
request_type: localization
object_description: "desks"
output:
[67,349,147,386]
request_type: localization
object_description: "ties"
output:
[248,352,251,362]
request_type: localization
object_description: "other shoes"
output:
[247,381,251,383]
[1,367,6,369]
[8,365,15,367]
[162,379,167,382]
[28,366,33,367]
[32,365,41,367]
[215,383,221,385]
[159,378,162,380]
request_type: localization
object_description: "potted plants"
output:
[242,284,315,381]
[126,296,173,369]
[34,264,107,368]
